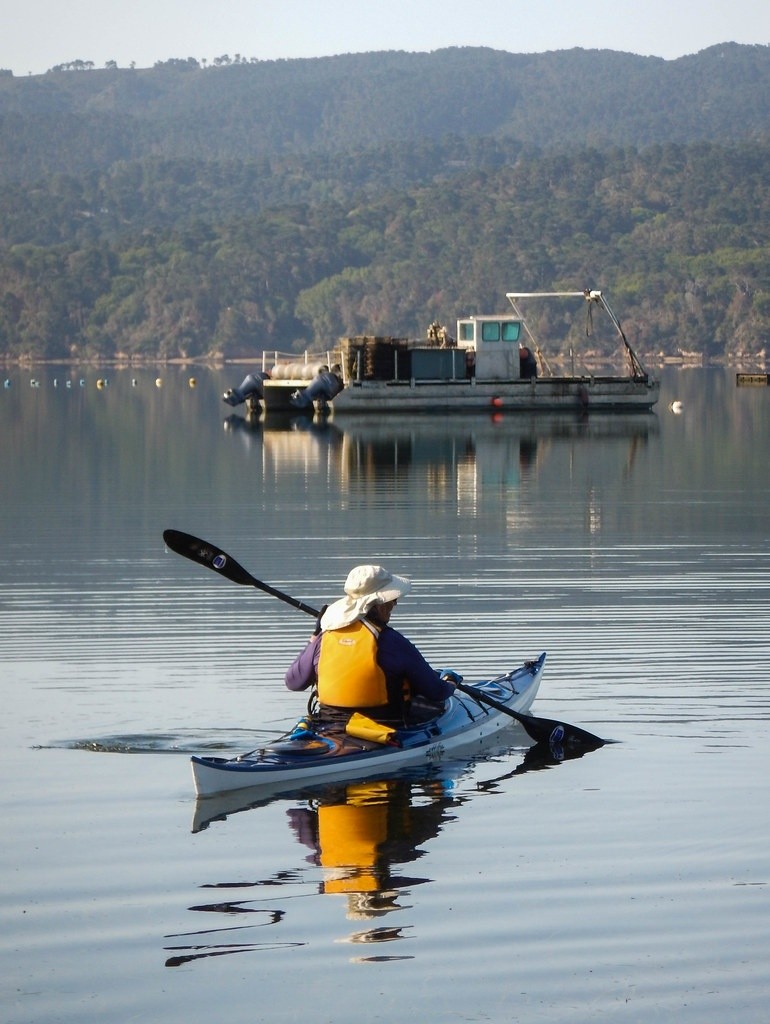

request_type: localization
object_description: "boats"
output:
[217,288,664,415]
[187,646,548,806]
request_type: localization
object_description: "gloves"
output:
[314,606,326,634]
[440,668,463,687]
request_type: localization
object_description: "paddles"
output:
[162,528,607,755]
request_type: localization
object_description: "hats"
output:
[321,565,411,630]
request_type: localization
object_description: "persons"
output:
[285,566,463,723]
[520,346,539,379]
[287,783,455,921]
